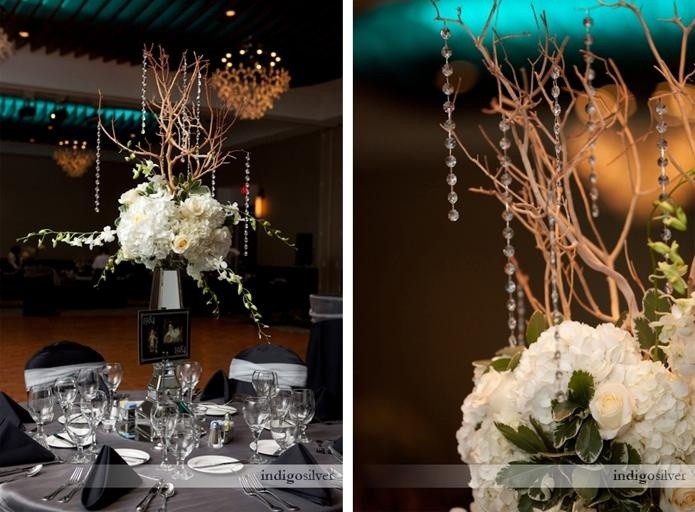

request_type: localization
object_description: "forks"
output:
[41,465,97,505]
[237,473,299,511]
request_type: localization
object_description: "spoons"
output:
[159,483,177,512]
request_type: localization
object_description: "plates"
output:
[187,455,245,474]
[112,447,149,468]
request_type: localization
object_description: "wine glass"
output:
[26,364,319,464]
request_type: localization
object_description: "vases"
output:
[136,265,192,419]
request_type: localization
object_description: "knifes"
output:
[134,480,167,512]
[0,459,63,485]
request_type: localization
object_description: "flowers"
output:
[13,41,300,346]
[430,0,695,512]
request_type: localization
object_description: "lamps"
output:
[213,28,292,121]
[54,136,95,179]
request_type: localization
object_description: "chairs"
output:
[229,342,307,396]
[23,340,106,399]
